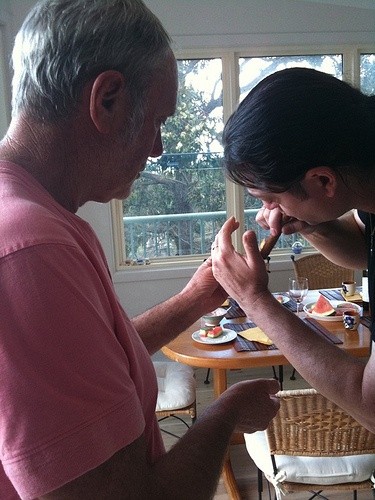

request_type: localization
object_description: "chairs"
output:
[244,386,375,500]
[153,361,198,439]
[289,247,355,291]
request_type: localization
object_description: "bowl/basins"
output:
[303,300,361,321]
[201,308,227,326]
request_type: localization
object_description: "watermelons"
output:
[333,303,360,316]
[311,294,336,316]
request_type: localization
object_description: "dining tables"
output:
[159,286,373,500]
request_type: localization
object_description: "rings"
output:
[213,245,218,250]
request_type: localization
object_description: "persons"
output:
[211,67,375,433]
[0,0,280,500]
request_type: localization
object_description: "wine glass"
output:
[288,277,309,320]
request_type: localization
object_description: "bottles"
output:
[362,269,369,311]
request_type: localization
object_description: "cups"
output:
[342,280,357,296]
[343,310,360,330]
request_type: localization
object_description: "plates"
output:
[273,295,290,304]
[191,329,237,344]
